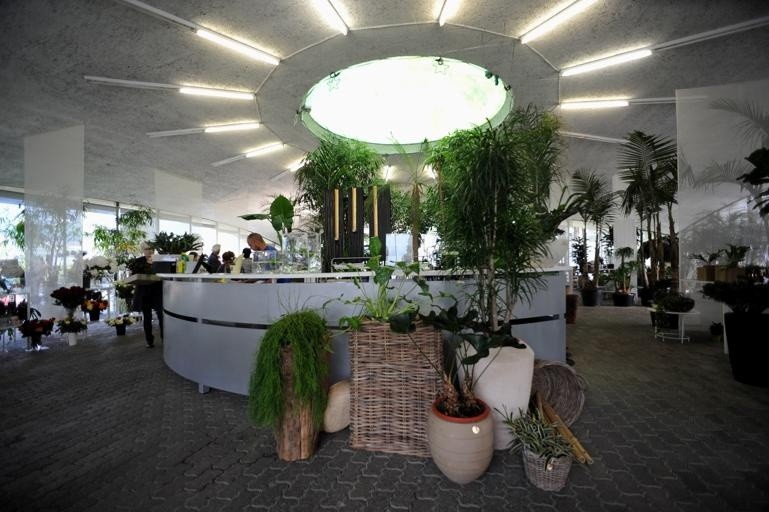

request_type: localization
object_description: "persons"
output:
[189,251,204,273]
[207,244,221,273]
[241,248,252,272]
[129,240,163,348]
[216,251,244,273]
[246,234,279,272]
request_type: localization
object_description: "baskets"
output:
[522,447,572,492]
[348,320,444,457]
[529,358,588,427]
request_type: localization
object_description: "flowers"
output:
[79,256,119,279]
[55,318,88,334]
[81,293,110,313]
[50,286,86,317]
[106,315,133,326]
[19,319,56,348]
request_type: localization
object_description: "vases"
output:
[116,325,127,336]
[89,308,101,321]
[67,332,78,346]
[151,232,203,274]
[63,317,74,325]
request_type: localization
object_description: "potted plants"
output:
[710,322,723,345]
[660,137,680,297]
[649,303,678,329]
[613,248,634,306]
[420,102,601,452]
[389,307,524,486]
[717,240,750,283]
[635,131,669,302]
[658,294,695,312]
[690,249,720,280]
[616,129,657,307]
[702,280,768,389]
[568,172,625,307]
[248,290,338,462]
[686,299,688,299]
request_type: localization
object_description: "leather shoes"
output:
[146,344,154,348]
